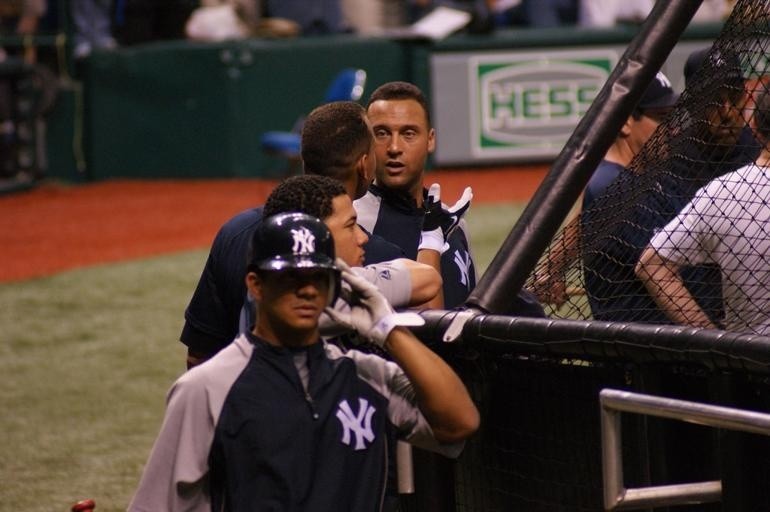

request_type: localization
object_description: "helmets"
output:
[247,209,340,278]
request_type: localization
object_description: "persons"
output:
[0,0,116,55]
[636,83,770,327]
[583,73,675,322]
[342,0,418,38]
[185,0,300,41]
[239,174,443,332]
[523,50,761,305]
[126,212,480,512]
[179,101,473,372]
[352,83,475,312]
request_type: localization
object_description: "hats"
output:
[683,47,752,97]
[636,69,684,109]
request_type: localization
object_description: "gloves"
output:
[323,257,427,350]
[416,183,475,254]
